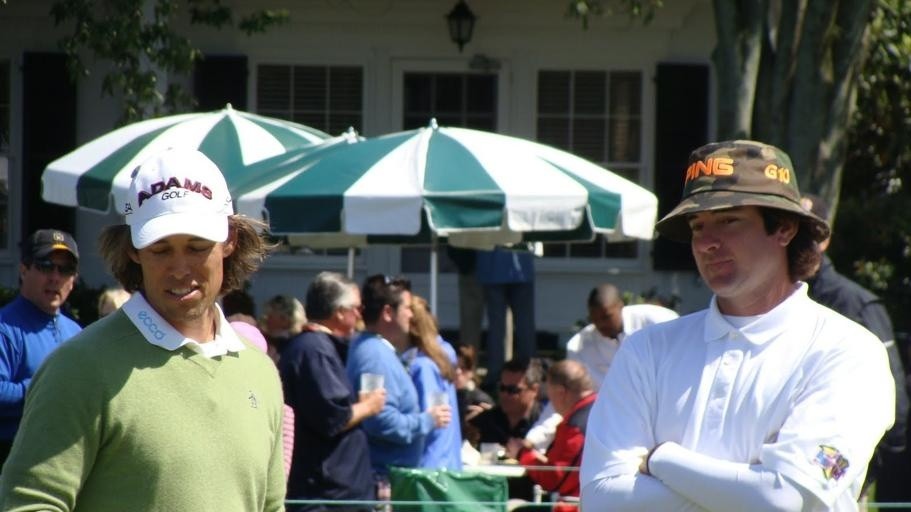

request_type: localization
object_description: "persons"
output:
[577,134,902,512]
[800,197,910,505]
[2,229,132,464]
[498,281,680,512]
[1,144,290,512]
[222,271,496,512]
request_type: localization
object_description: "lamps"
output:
[442,1,479,55]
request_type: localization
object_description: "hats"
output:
[109,147,235,250]
[23,229,79,265]
[656,140,830,245]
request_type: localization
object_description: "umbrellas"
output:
[38,100,334,215]
[226,125,370,280]
[231,117,658,321]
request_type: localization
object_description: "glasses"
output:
[33,258,77,276]
[498,383,522,393]
[382,279,396,291]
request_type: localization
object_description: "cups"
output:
[425,389,450,429]
[360,371,384,401]
[480,442,500,465]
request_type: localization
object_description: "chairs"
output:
[386,461,509,510]
